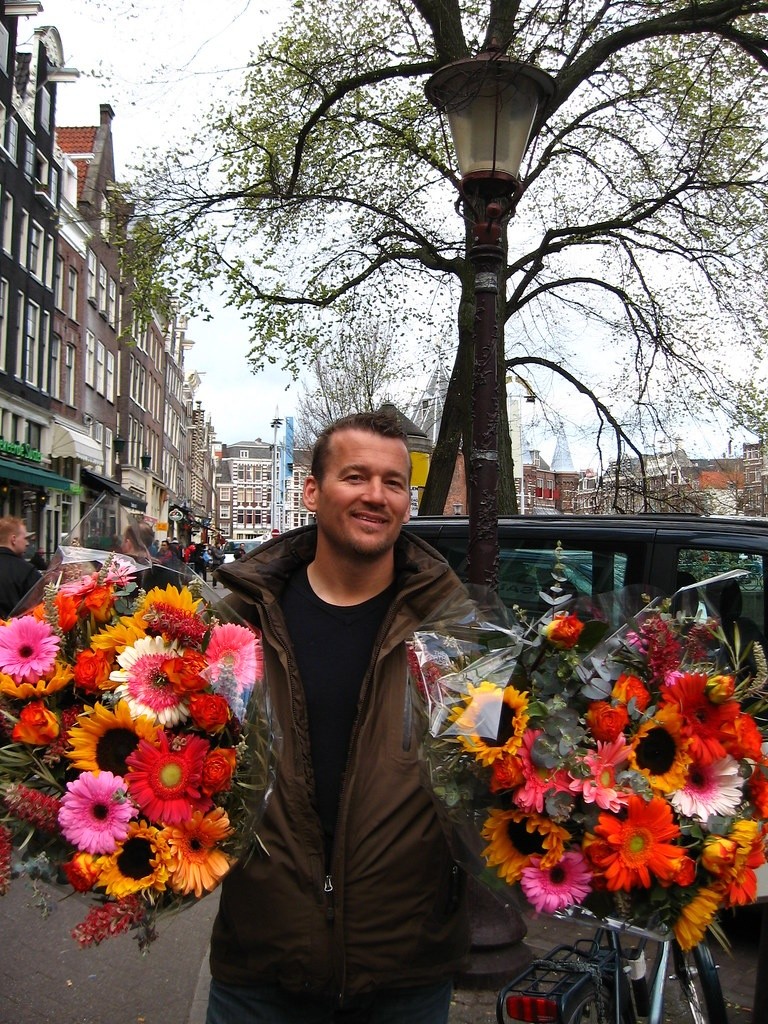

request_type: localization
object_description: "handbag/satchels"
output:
[203,552,211,562]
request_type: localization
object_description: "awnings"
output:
[0,459,75,491]
[51,424,106,467]
[80,469,148,513]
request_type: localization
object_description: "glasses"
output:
[161,544,166,546]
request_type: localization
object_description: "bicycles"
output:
[680,560,765,592]
[496,890,728,1024]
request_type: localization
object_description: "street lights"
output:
[415,45,559,586]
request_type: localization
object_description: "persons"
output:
[30,547,48,571]
[106,520,247,588]
[0,514,44,621]
[198,411,483,1024]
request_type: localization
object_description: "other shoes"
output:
[213,586,217,589]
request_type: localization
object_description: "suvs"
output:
[400,512,768,735]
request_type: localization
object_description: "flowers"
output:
[405,539,768,966]
[0,549,279,952]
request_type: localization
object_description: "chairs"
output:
[704,579,768,687]
[670,571,698,627]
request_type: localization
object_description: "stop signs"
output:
[271,529,280,538]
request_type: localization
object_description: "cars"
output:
[221,538,262,564]
[496,549,628,632]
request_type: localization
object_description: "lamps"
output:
[126,441,151,472]
[85,416,125,454]
